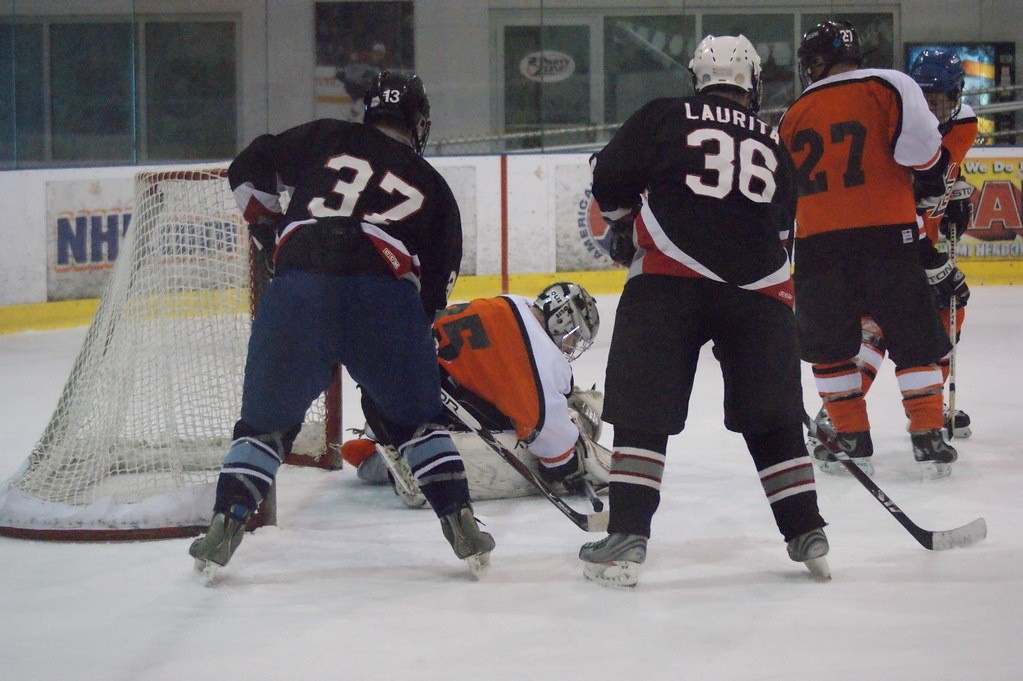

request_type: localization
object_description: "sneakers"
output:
[802,404,837,454]
[187,513,246,588]
[577,533,649,589]
[911,430,959,481]
[905,402,972,439]
[814,431,875,475]
[788,529,832,581]
[438,509,494,580]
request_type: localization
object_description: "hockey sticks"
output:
[802,412,987,552]
[582,478,607,515]
[439,387,611,533]
[948,177,959,443]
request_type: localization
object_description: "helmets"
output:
[797,20,864,80]
[364,69,432,138]
[911,47,965,126]
[536,281,602,360]
[687,34,764,94]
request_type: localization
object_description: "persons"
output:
[579,34,828,564]
[340,281,612,510]
[778,18,978,463]
[188,71,496,568]
